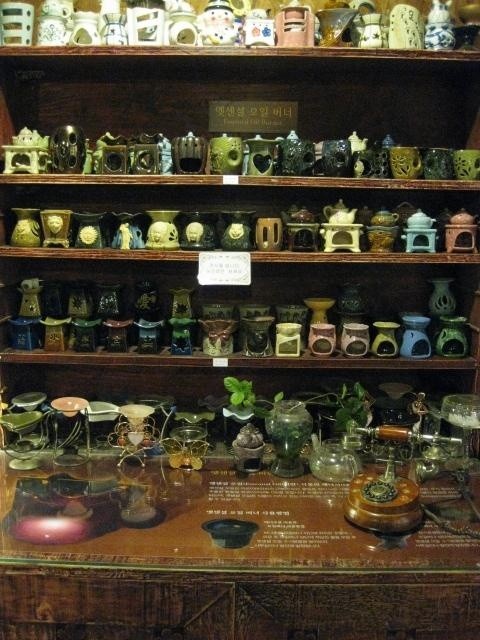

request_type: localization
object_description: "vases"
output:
[0,0,480,546]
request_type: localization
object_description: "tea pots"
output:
[285,197,480,229]
[347,130,368,153]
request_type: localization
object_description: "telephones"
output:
[342,419,464,534]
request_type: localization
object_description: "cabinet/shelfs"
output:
[0,559,480,640]
